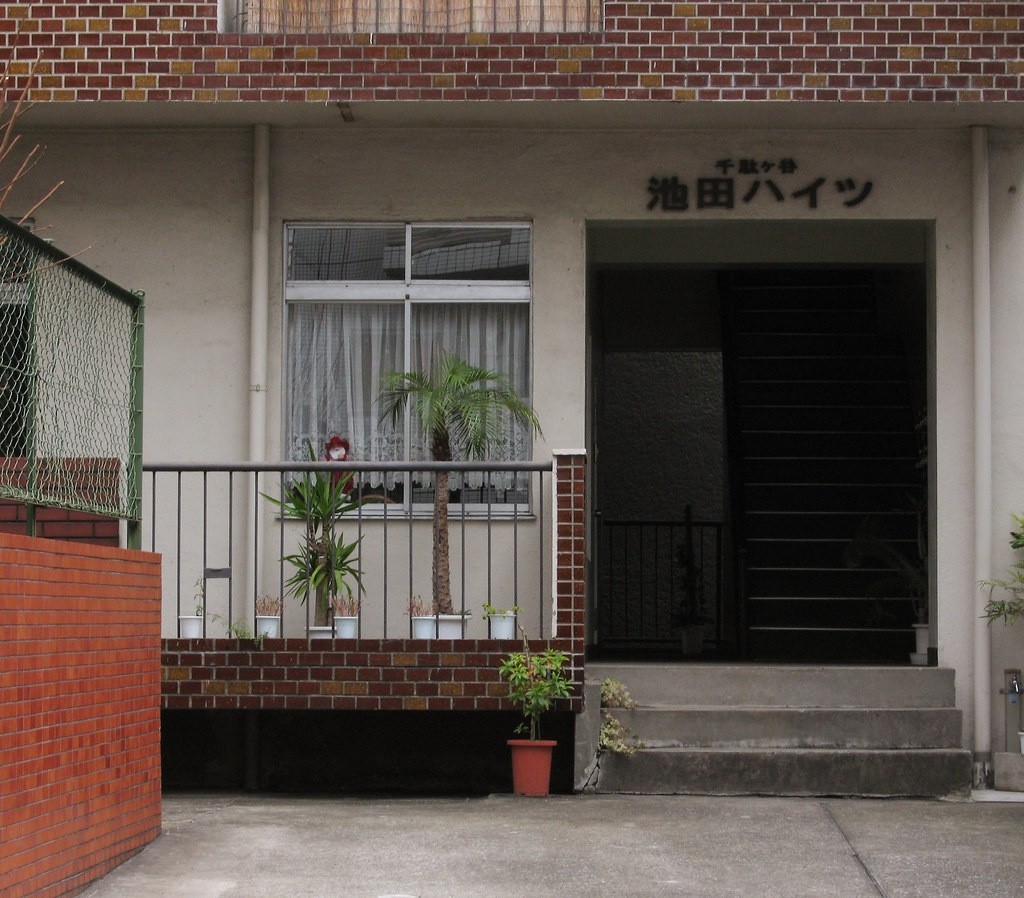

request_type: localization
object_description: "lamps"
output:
[326,436,355,497]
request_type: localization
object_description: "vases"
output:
[254,616,283,638]
[332,616,363,638]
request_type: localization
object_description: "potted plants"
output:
[259,437,397,639]
[497,623,579,800]
[179,577,269,639]
[973,509,1024,754]
[850,484,928,666]
[481,602,525,639]
[374,347,546,639]
